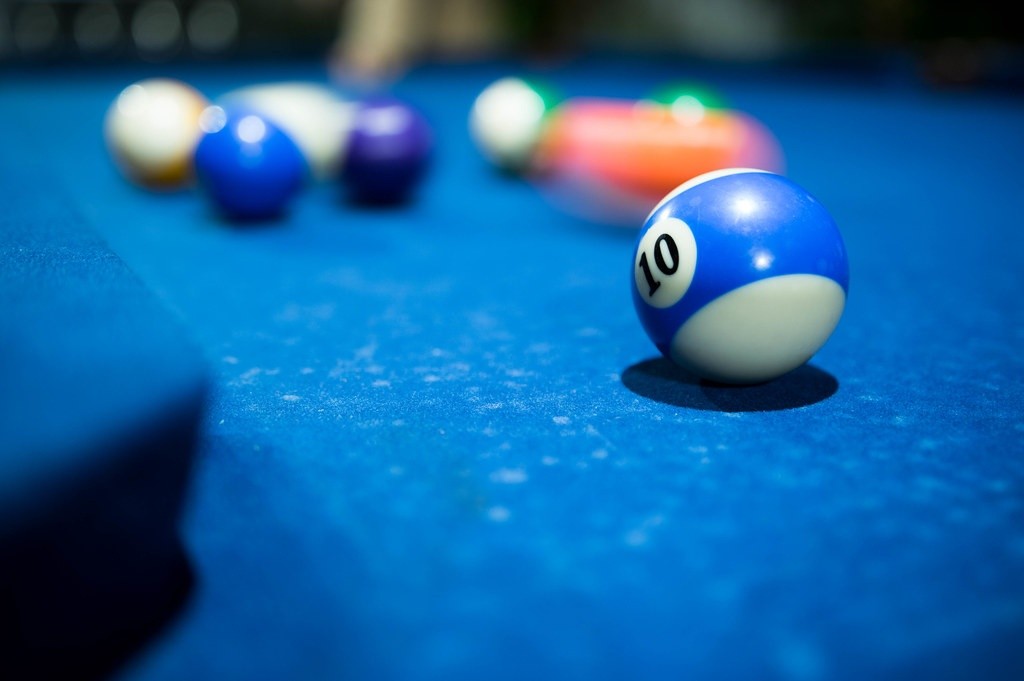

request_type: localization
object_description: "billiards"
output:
[629,166,854,380]
[102,70,778,224]
